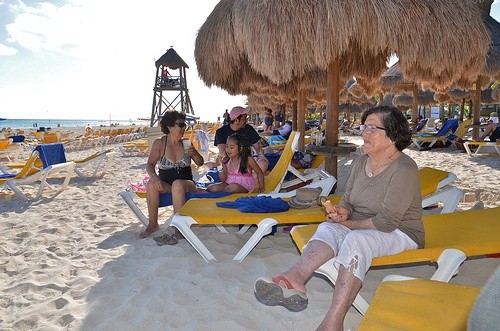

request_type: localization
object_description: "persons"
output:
[264,109,272,131]
[223,109,229,119]
[261,121,292,137]
[214,106,269,173]
[207,134,265,193]
[163,69,170,83]
[473,121,500,142]
[141,111,205,239]
[254,106,426,331]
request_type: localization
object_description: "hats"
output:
[230,106,250,120]
[287,188,321,209]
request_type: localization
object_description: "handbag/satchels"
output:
[216,196,290,214]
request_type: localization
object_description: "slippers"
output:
[255,275,309,312]
[153,234,178,246]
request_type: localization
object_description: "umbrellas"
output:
[194,0,500,196]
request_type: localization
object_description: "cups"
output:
[316,134,323,146]
[183,140,191,156]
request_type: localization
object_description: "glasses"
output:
[360,124,389,133]
[174,122,186,128]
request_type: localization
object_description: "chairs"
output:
[0,116,500,331]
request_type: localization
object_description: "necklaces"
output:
[368,172,373,177]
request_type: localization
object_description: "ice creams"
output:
[322,200,336,213]
